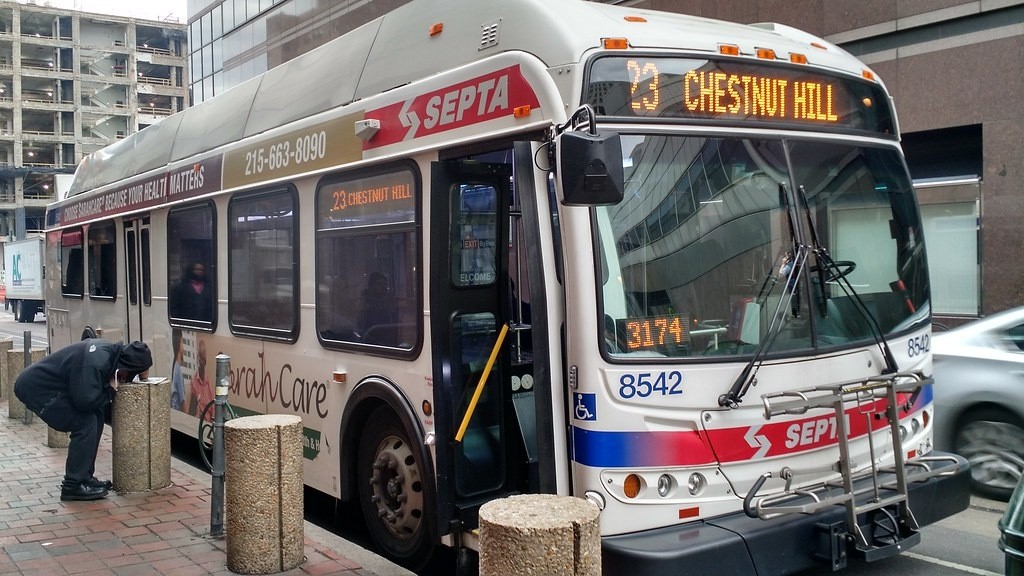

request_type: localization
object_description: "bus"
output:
[40,0,974,576]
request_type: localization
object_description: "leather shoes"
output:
[60,483,108,500]
[85,476,113,488]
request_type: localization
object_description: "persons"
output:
[174,260,210,322]
[778,250,801,321]
[363,271,390,303]
[13,338,152,501]
[168,327,214,423]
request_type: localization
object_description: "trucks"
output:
[3,235,46,323]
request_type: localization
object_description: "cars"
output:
[930,305,1024,503]
